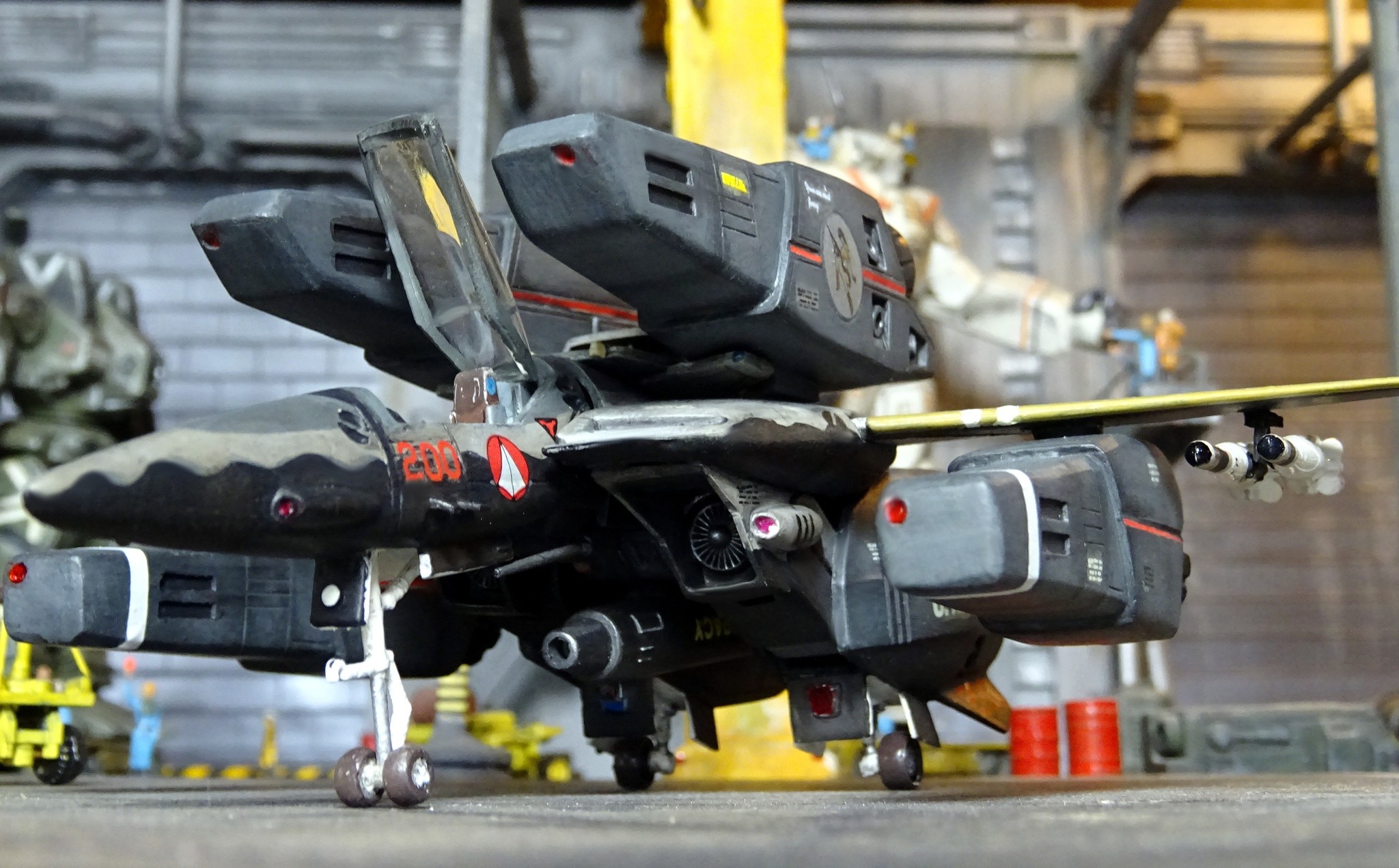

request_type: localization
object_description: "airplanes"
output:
[1,105,1399,811]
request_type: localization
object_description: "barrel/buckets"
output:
[1008,708,1060,777]
[1065,699,1121,775]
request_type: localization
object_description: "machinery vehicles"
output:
[1,617,99,786]
